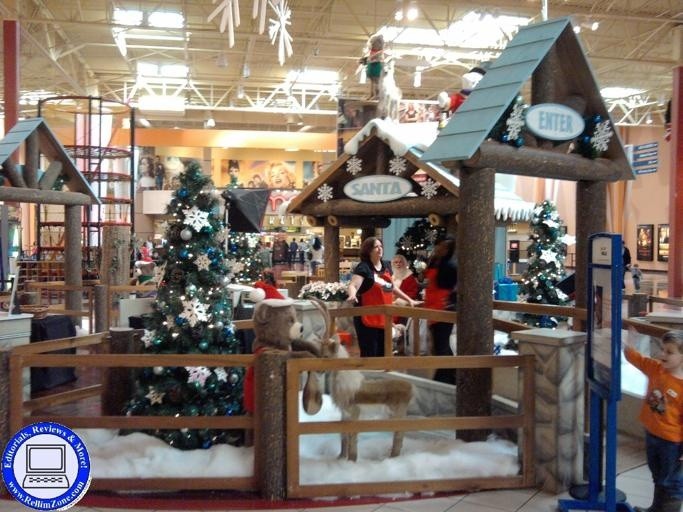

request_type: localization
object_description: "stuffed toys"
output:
[127,255,162,299]
[244,279,324,447]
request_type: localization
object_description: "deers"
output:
[308,287,414,464]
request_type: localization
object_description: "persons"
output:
[138,156,172,190]
[424,236,461,387]
[346,236,424,357]
[388,255,419,325]
[621,239,632,289]
[622,328,683,512]
[225,157,292,189]
[628,263,642,291]
[288,237,325,272]
